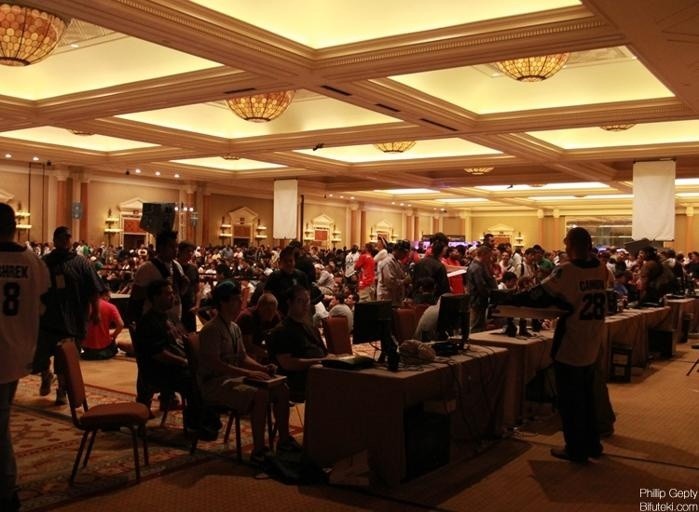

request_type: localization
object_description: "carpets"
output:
[8,371,304,511]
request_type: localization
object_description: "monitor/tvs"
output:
[351,300,399,361]
[437,294,471,347]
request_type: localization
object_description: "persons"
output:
[2,204,53,510]
[24,224,699,479]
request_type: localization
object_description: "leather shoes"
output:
[551,448,587,463]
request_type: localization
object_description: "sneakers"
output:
[160,400,183,410]
[55,389,65,405]
[40,373,53,395]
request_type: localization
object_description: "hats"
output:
[205,281,235,305]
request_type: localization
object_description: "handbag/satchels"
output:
[399,339,435,363]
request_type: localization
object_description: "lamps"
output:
[488,52,571,83]
[0,0,68,67]
[224,88,296,124]
[222,155,241,161]
[73,128,96,135]
[372,141,416,152]
[463,166,495,175]
[598,123,635,130]
